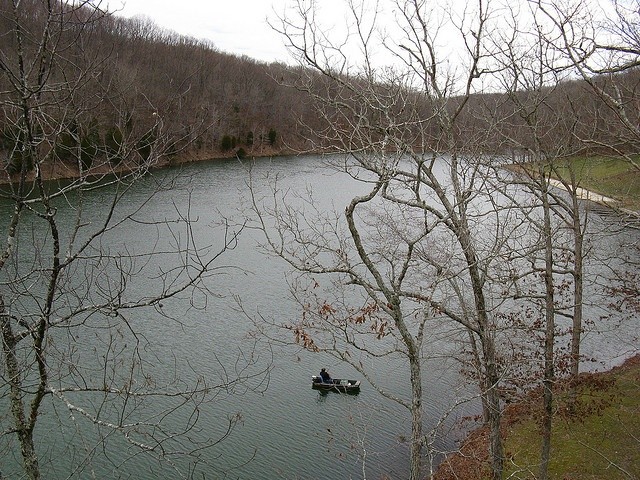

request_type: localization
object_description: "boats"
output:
[312,375,361,389]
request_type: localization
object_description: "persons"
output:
[319,368,333,384]
